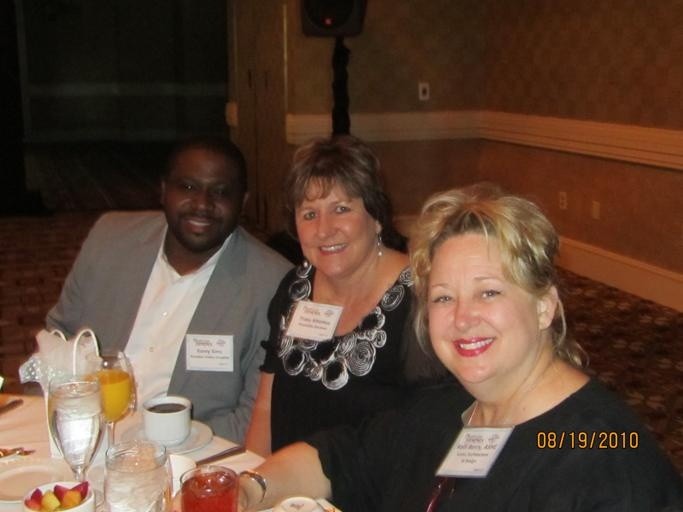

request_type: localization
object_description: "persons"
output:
[42,123,296,448]
[163,180,683,512]
[246,133,419,462]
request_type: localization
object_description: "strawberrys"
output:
[23,488,43,510]
[71,481,89,501]
[53,486,68,502]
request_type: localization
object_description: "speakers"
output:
[303,0,364,36]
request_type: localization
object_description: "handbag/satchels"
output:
[40,328,109,463]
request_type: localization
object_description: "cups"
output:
[141,393,194,447]
[22,479,96,512]
[103,439,176,511]
[179,464,240,511]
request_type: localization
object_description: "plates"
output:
[120,418,214,455]
[0,464,63,504]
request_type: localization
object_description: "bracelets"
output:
[238,469,268,504]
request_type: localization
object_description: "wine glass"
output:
[44,347,135,508]
